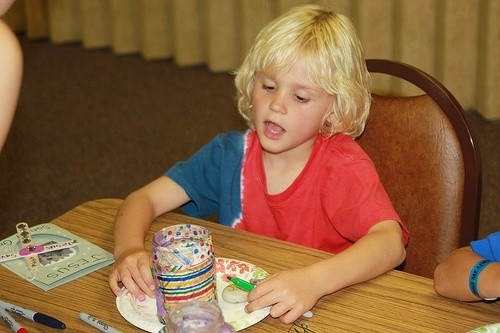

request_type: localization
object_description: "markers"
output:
[0,299,67,330]
[225,274,314,320]
[79,310,126,333]
[0,306,30,333]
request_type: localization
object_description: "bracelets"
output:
[468,259,498,302]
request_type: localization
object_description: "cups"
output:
[151,223,218,325]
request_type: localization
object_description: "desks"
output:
[0,198,500,333]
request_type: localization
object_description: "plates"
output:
[116,256,272,333]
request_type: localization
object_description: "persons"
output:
[433,230,500,302]
[108,4,409,324]
[0,16,24,151]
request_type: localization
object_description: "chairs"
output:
[354,59,482,279]
